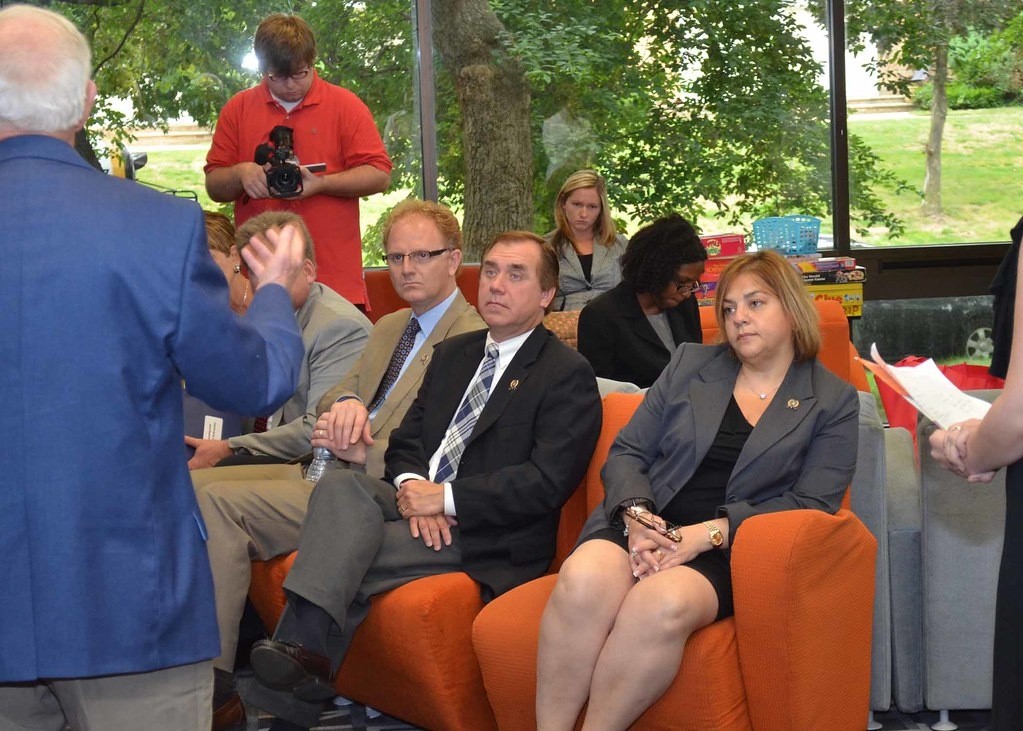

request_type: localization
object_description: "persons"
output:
[233,230,603,731]
[184,200,489,731]
[577,213,708,389]
[185,210,374,469]
[204,13,393,311]
[929,217,1023,731]
[542,169,629,311]
[0,5,304,731]
[535,251,861,730]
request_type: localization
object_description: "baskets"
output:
[750,214,823,255]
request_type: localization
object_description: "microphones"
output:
[255,143,270,165]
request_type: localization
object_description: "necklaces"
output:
[741,366,767,399]
[239,279,248,312]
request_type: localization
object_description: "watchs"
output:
[702,521,724,549]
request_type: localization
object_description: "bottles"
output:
[306,447,337,484]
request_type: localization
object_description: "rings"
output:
[654,549,664,560]
[401,505,405,511]
[633,554,637,560]
[319,430,322,438]
[953,425,961,430]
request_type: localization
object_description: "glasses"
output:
[668,277,702,292]
[265,62,310,82]
[382,247,450,265]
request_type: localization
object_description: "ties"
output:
[367,317,422,413]
[433,343,500,488]
[253,417,269,433]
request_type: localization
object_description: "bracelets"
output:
[623,511,649,536]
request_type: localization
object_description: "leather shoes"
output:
[211,689,247,731]
[249,638,338,703]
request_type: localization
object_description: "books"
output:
[695,232,866,307]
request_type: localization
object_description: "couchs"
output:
[244,264,1006,731]
